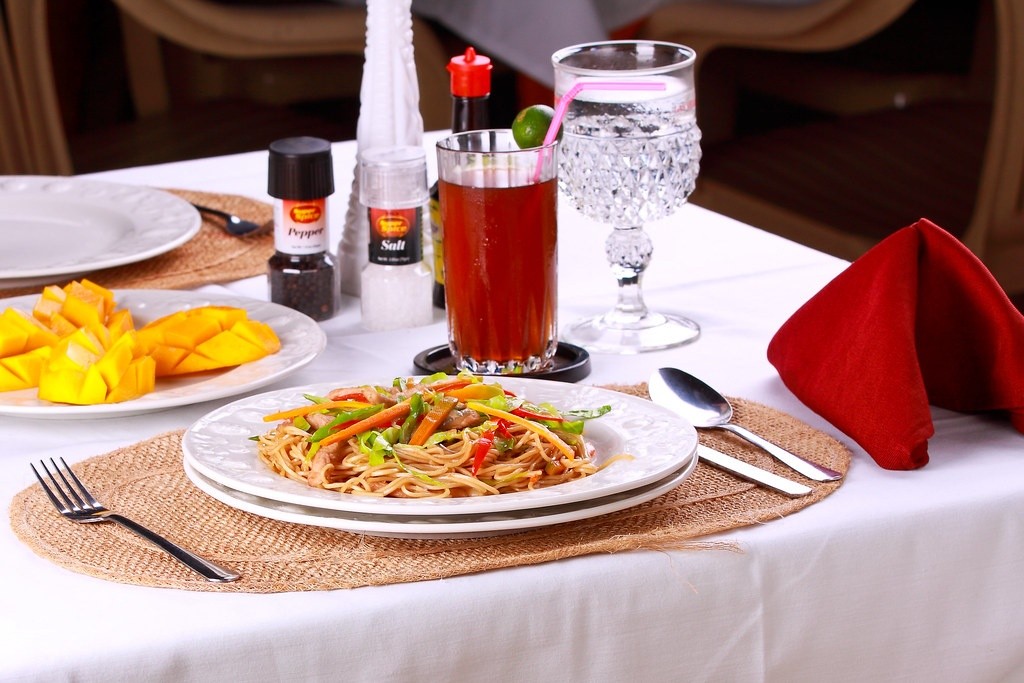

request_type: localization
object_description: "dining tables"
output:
[0,128,1022,683]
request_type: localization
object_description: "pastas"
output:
[256,422,635,499]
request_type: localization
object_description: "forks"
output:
[189,202,274,238]
[29,456,238,584]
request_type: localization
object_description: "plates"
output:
[0,290,328,419]
[0,174,202,289]
[182,375,700,539]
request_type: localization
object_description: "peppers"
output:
[249,369,576,476]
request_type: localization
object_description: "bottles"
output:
[430,46,493,312]
[267,136,342,322]
[359,145,433,333]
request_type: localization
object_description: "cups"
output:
[435,127,559,376]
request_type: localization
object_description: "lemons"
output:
[512,105,563,149]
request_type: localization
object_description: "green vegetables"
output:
[520,404,611,435]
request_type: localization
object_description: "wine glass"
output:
[550,39,702,356]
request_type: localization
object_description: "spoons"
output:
[647,367,845,483]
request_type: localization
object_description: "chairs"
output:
[689,0,1023,306]
[1,2,448,181]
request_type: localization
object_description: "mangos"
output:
[0,279,281,405]
